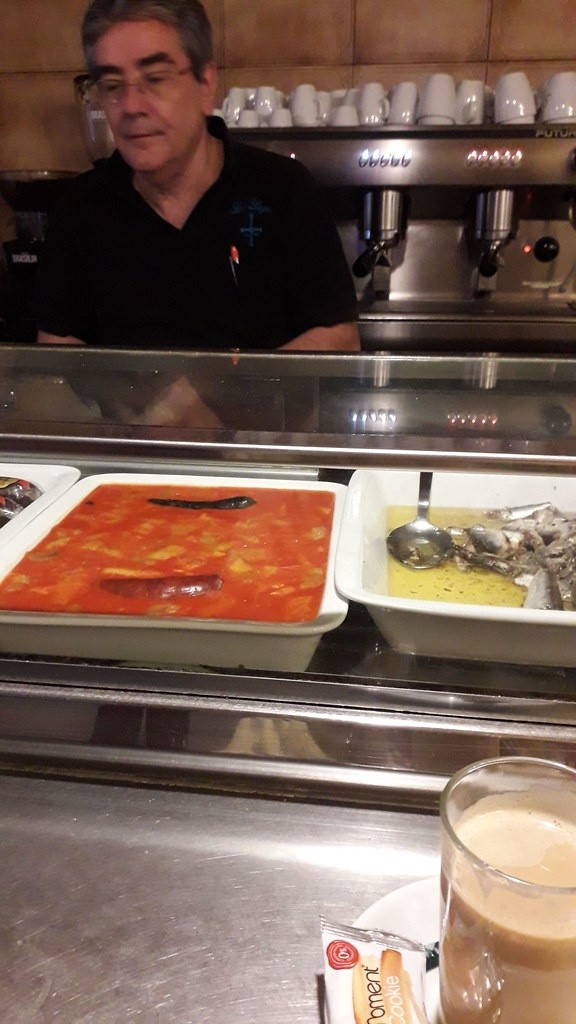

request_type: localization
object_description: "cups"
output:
[213,71,576,128]
[435,756,576,1024]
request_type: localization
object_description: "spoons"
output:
[386,472,454,570]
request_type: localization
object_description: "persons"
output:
[0,0,364,438]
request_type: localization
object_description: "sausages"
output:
[98,575,224,598]
[148,495,256,509]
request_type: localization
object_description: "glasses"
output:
[87,64,193,105]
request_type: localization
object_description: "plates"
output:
[324,874,441,1024]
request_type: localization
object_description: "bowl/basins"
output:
[333,469,575,668]
[0,463,83,544]
[0,473,351,673]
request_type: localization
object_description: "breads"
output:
[351,949,425,1024]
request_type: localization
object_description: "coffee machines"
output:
[74,74,117,168]
